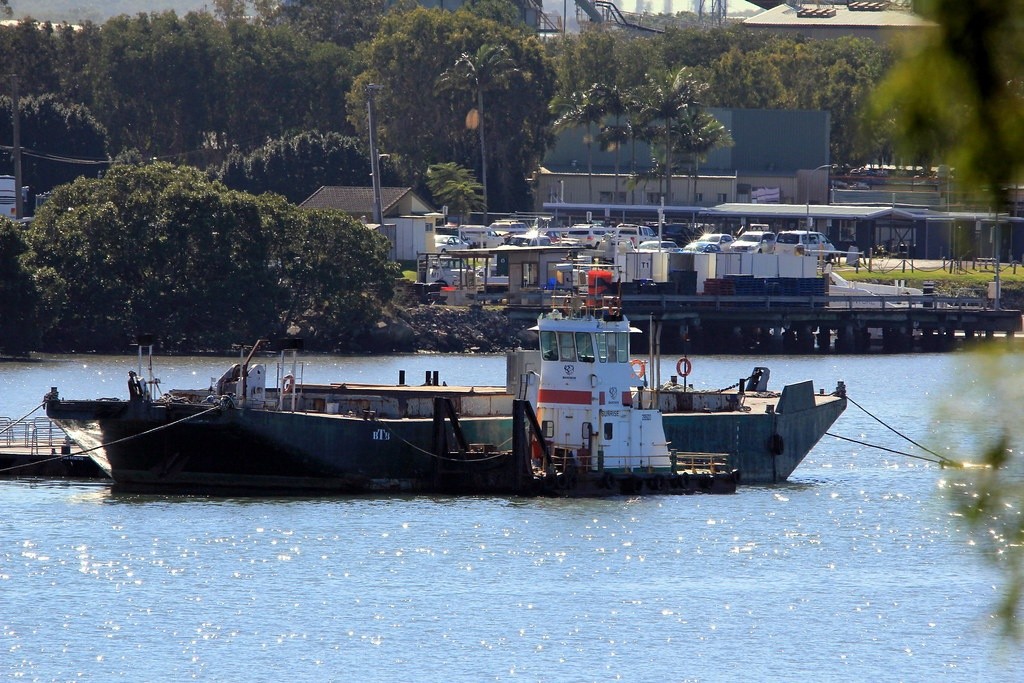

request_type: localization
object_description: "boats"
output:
[41,263,847,496]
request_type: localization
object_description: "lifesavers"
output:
[282,375,294,394]
[630,359,645,378]
[676,357,692,378]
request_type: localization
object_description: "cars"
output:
[434,223,838,297]
